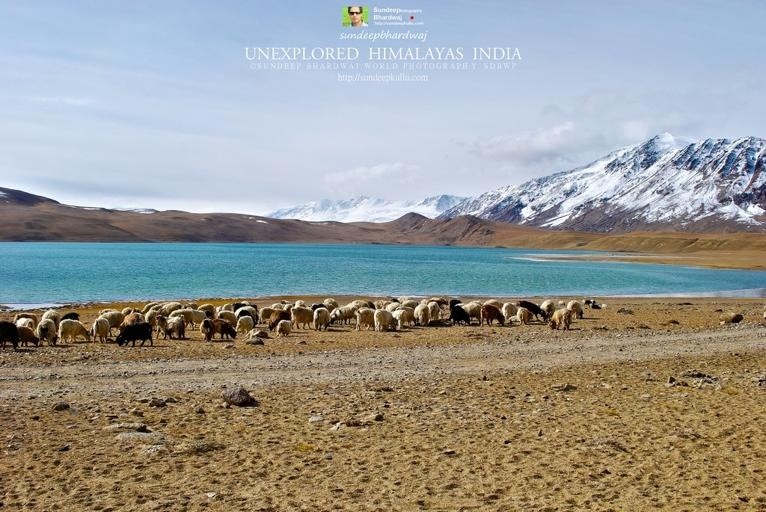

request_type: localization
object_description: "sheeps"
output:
[0,296,602,351]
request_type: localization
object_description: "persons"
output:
[348,7,368,27]
